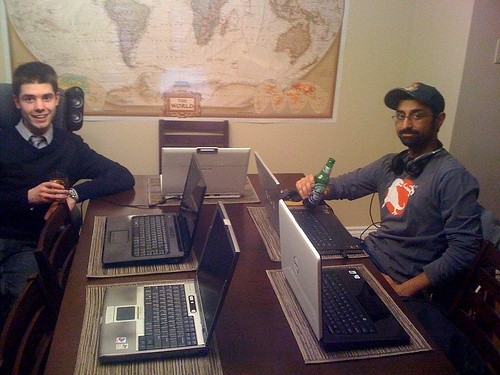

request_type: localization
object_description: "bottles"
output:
[302,158,336,212]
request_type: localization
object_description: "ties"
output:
[30,137,45,148]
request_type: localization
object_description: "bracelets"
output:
[67,188,80,202]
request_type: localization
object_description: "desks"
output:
[46,173,458,372]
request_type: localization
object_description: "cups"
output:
[47,172,70,201]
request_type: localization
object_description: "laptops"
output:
[101,153,207,267]
[254,150,363,255]
[278,199,410,352]
[159,147,251,199]
[98,201,240,365]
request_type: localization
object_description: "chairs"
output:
[0,270,59,375]
[449,239,500,375]
[158,119,229,173]
[0,83,66,135]
[434,203,495,314]
[34,199,80,301]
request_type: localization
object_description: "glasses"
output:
[391,113,437,123]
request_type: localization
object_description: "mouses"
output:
[279,188,302,202]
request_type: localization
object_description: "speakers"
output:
[65,87,84,131]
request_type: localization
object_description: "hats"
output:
[384,81,444,114]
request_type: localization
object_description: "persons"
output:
[0,61,135,314]
[295,81,482,300]
